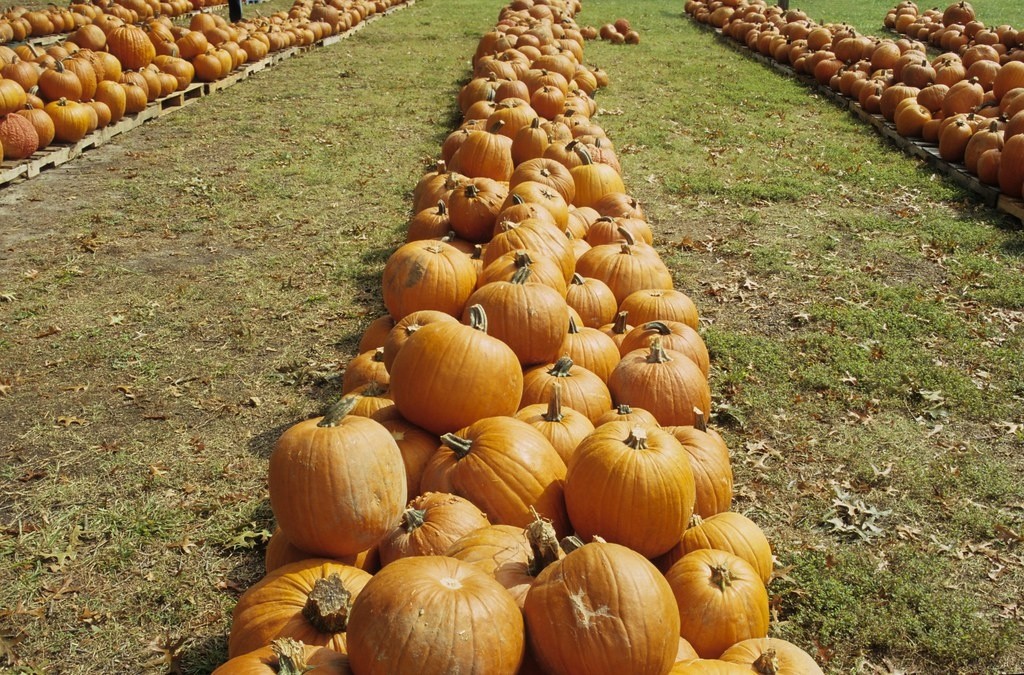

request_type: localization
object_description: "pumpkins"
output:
[208,0,825,675]
[683,0,1024,203]
[0,0,406,167]
